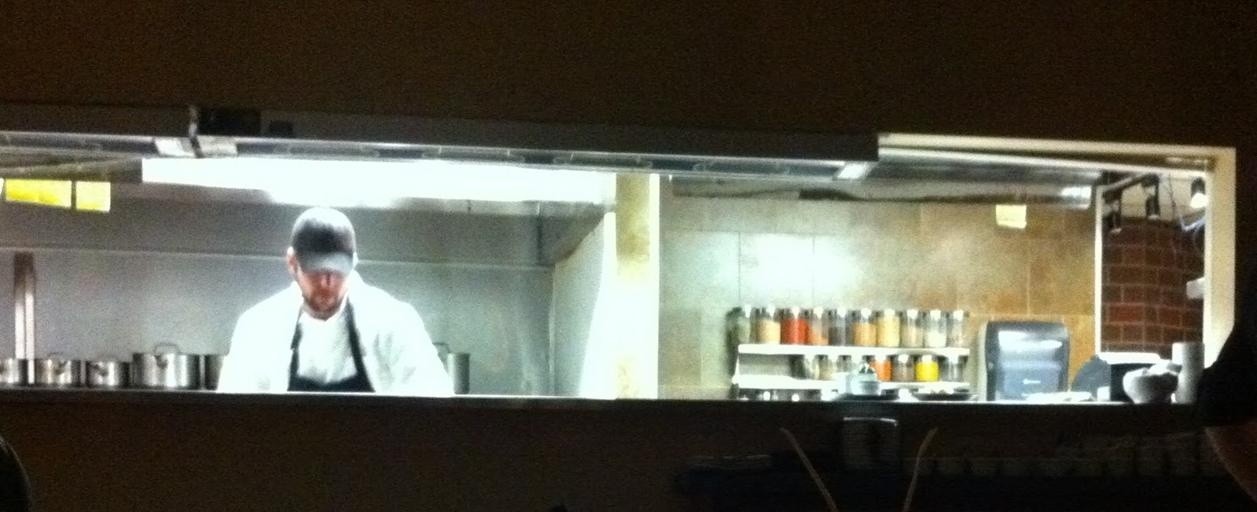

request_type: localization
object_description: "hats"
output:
[289,207,357,278]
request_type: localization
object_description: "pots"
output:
[1,338,229,389]
[430,340,470,395]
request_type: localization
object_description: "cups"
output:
[851,381,882,395]
[1172,341,1205,403]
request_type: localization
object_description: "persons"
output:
[216,205,456,400]
[1192,267,1256,503]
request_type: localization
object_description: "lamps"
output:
[1100,170,1208,235]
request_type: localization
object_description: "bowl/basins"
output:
[1122,375,1177,405]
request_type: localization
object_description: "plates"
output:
[843,392,900,400]
[909,391,979,403]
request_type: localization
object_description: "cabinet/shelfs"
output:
[731,342,972,402]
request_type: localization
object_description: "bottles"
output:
[730,306,969,380]
